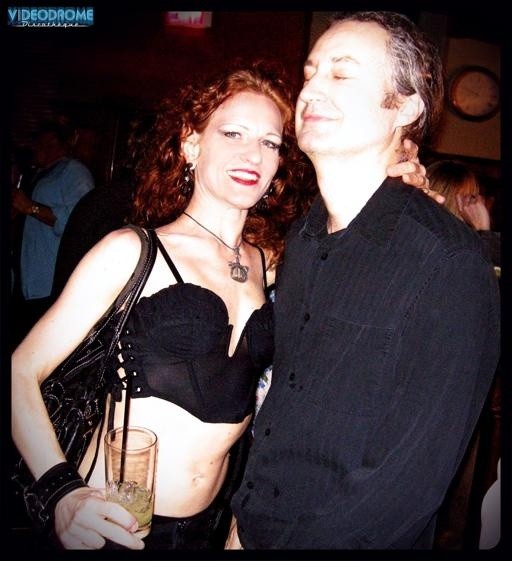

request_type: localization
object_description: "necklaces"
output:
[179,208,250,283]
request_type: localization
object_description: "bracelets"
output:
[31,202,41,217]
[23,461,91,530]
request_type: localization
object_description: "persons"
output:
[217,13,502,550]
[10,51,450,552]
[424,159,502,551]
[49,109,155,312]
[10,119,96,351]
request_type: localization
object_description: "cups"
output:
[10,163,29,190]
[103,424,158,541]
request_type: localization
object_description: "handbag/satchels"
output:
[10,223,148,513]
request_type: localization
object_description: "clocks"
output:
[443,66,500,123]
